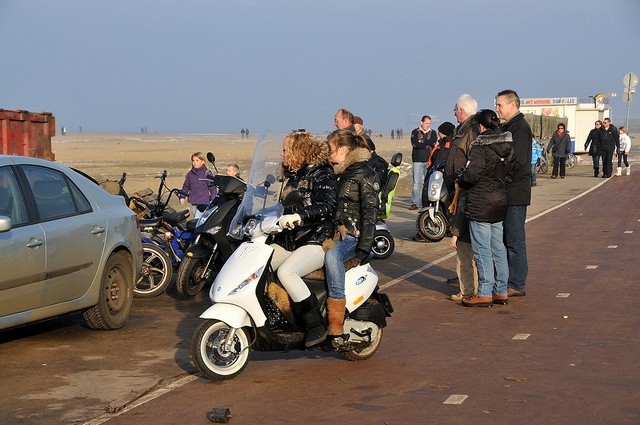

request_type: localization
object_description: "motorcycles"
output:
[176,152,280,301]
[191,131,393,381]
[118,172,172,299]
[127,197,199,276]
[417,161,452,242]
[370,153,403,259]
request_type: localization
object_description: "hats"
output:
[438,121,455,135]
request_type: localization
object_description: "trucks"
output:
[0,109,55,163]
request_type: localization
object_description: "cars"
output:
[0,153,144,330]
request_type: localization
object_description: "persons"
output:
[444,93,481,202]
[584,120,602,177]
[365,140,389,191]
[325,130,382,336]
[598,118,619,178]
[455,109,516,309]
[448,190,480,302]
[353,116,371,140]
[225,163,244,181]
[364,128,404,139]
[531,135,542,186]
[495,88,533,298]
[547,123,571,178]
[412,122,456,243]
[409,115,438,210]
[333,108,356,134]
[178,152,217,218]
[269,128,337,348]
[614,127,633,177]
[241,127,250,138]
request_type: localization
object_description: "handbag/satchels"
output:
[552,134,567,153]
[257,290,291,333]
[265,265,295,325]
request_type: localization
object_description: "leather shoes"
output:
[494,294,508,305]
[462,294,492,307]
[507,288,526,295]
[410,204,417,210]
[602,173,612,178]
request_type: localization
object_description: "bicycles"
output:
[154,170,210,233]
[564,153,577,168]
[534,144,548,174]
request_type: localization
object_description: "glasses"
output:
[558,128,564,129]
[603,122,607,125]
[595,124,599,125]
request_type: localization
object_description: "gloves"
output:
[278,212,302,230]
[355,248,369,258]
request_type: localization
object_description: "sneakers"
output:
[451,292,472,301]
[551,175,557,179]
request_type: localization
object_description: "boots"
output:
[327,297,346,335]
[593,167,600,177]
[616,148,620,154]
[294,293,328,347]
[625,165,630,176]
[615,167,622,176]
